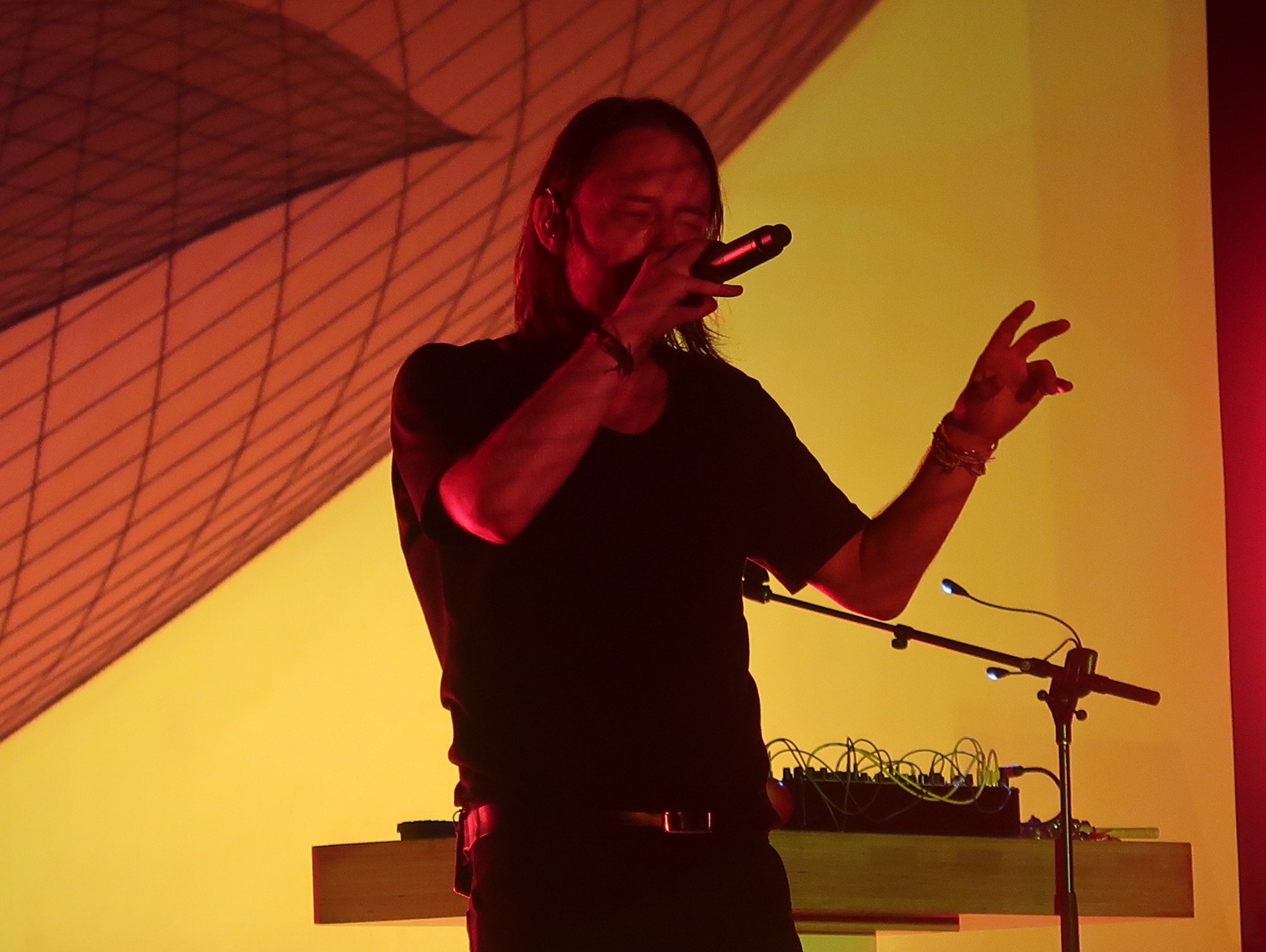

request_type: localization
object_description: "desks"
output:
[306,837,1198,952]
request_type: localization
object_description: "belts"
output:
[464,794,771,851]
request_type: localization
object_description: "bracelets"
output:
[583,323,637,380]
[928,409,1002,478]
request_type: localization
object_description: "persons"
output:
[386,92,1075,952]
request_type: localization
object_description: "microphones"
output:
[692,224,797,280]
